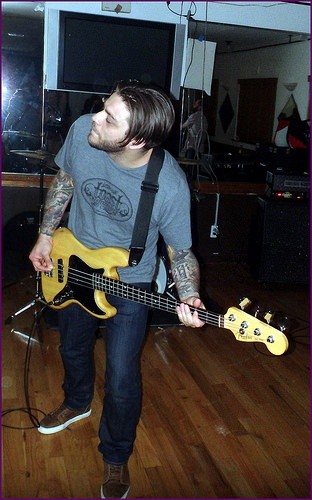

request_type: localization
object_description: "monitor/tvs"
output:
[57,10,176,92]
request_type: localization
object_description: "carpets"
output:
[43,293,225,330]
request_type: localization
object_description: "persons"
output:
[29,80,207,500]
[182,98,208,180]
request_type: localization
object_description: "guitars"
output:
[41,226,294,355]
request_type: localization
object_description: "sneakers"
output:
[101,462,130,499]
[38,401,91,435]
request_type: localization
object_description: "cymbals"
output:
[9,148,59,160]
[41,156,60,171]
[2,129,42,139]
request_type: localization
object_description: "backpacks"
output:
[271,116,310,175]
